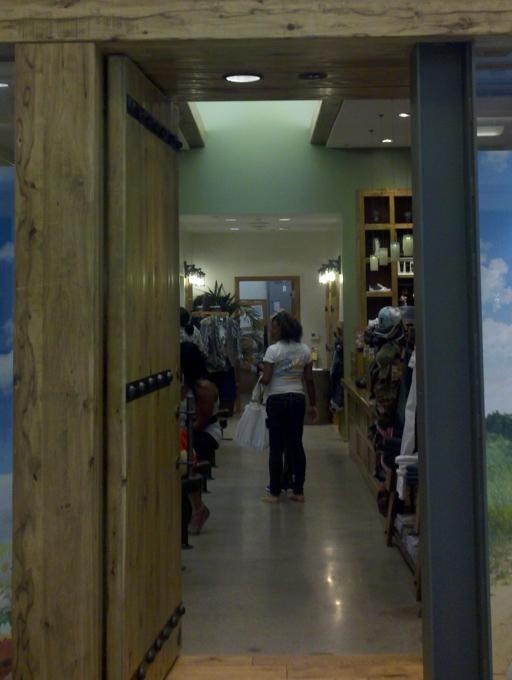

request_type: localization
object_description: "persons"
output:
[180,342,222,537]
[255,310,321,505]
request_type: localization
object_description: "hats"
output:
[374,306,402,339]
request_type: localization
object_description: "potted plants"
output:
[191,280,237,313]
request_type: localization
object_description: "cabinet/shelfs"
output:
[354,187,413,364]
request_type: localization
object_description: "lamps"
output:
[318,256,343,286]
[182,259,207,288]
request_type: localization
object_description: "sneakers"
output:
[368,283,391,293]
[261,483,304,504]
[188,504,209,534]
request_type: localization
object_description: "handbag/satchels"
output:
[235,385,270,454]
